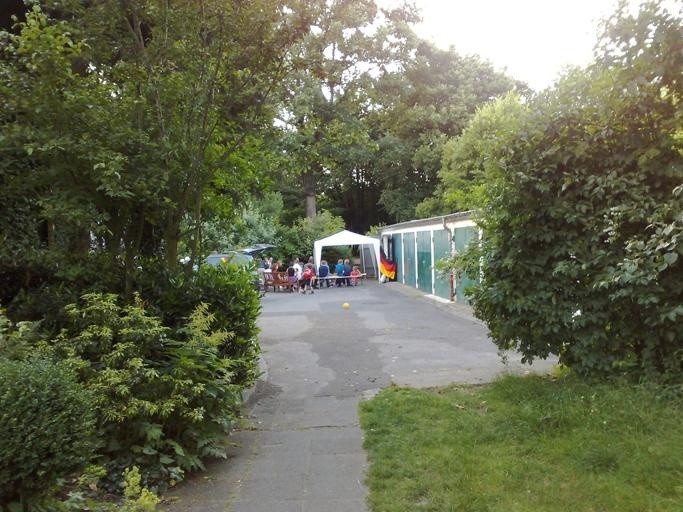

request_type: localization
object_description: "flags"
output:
[379,246,396,280]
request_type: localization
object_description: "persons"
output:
[335,258,345,287]
[319,260,333,288]
[253,255,316,294]
[350,266,362,286]
[343,259,352,286]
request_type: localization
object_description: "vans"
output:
[180,242,277,301]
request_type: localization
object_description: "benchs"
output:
[248,267,368,295]
